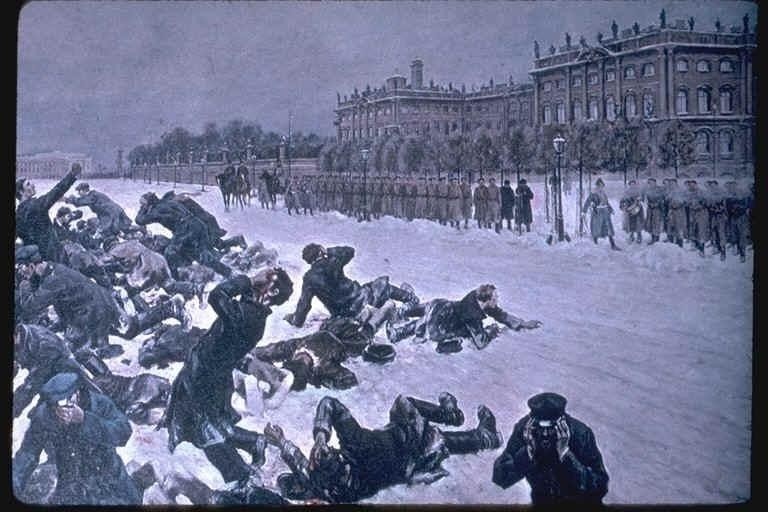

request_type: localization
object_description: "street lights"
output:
[171,160,179,188]
[156,160,162,185]
[355,146,379,223]
[545,131,574,246]
[143,161,148,182]
[200,157,208,193]
